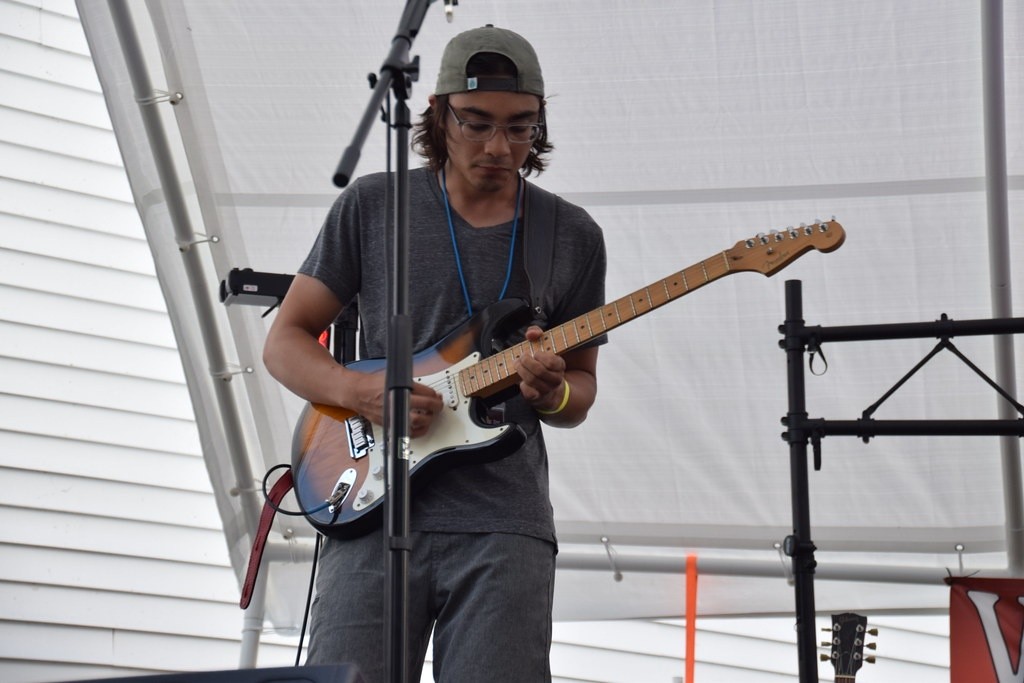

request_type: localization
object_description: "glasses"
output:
[447,100,543,144]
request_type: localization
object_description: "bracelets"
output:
[533,378,569,414]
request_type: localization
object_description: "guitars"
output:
[289,215,846,541]
[818,612,878,682]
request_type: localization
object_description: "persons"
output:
[265,26,606,683]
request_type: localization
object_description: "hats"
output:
[435,24,544,98]
